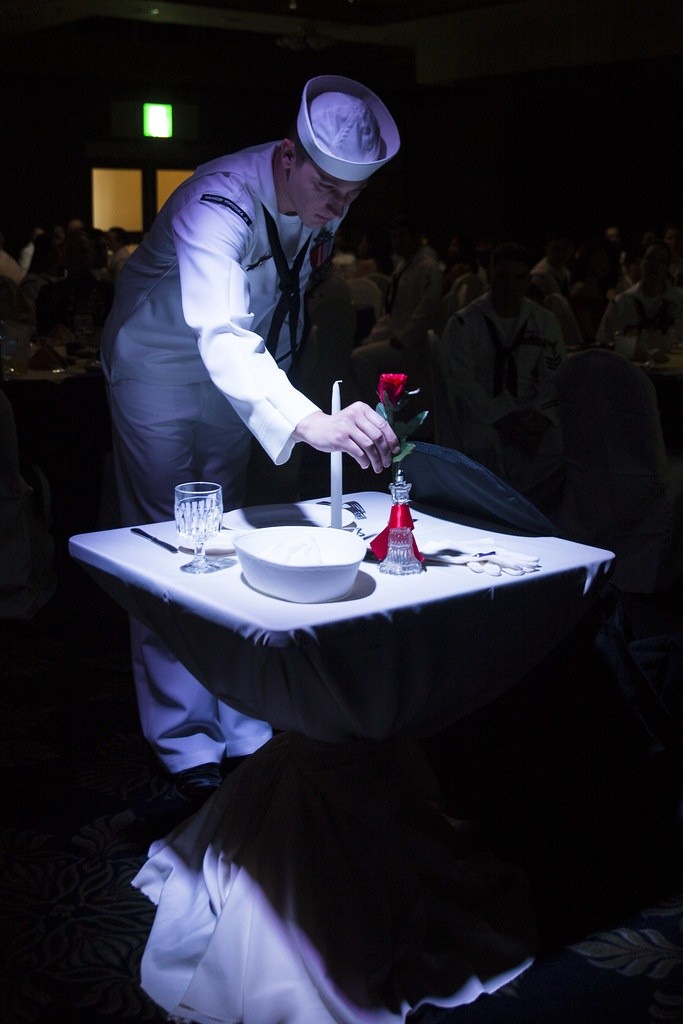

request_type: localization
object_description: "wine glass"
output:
[641,330,662,368]
[174,482,223,574]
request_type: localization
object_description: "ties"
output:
[260,200,314,383]
[482,313,529,399]
[633,296,668,331]
[383,264,409,315]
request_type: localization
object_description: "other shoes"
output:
[173,762,223,802]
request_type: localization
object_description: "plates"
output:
[178,529,235,555]
[223,504,355,529]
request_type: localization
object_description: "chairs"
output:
[0,272,683,596]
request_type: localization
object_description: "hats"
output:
[297,74,400,182]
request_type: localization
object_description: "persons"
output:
[440,242,569,509]
[101,75,401,797]
[0,218,132,356]
[328,224,683,411]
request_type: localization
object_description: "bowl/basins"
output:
[233,526,367,603]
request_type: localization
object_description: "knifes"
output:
[131,528,178,554]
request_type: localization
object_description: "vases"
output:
[368,484,424,576]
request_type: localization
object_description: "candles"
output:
[329,380,345,533]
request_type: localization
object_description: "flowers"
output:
[372,371,429,483]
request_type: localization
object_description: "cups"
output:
[0,320,31,377]
[614,336,638,359]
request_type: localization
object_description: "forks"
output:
[316,501,366,519]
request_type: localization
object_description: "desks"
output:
[602,341,683,378]
[0,336,103,381]
[68,489,617,975]
